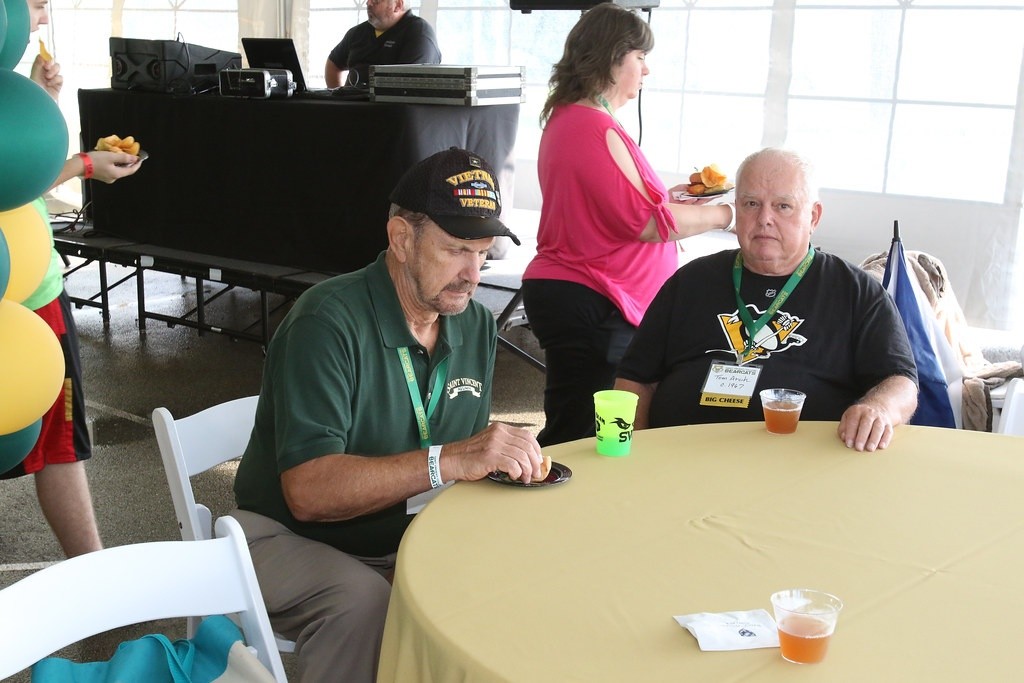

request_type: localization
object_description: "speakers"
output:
[110,37,242,97]
[509,0,659,14]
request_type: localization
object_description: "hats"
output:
[390,146,521,246]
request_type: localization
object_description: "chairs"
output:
[0,516,289,683]
[153,396,298,655]
[860,251,1023,432]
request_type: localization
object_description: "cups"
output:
[593,390,640,456]
[759,388,807,435]
[769,588,844,666]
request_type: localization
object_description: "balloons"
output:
[0,0,69,473]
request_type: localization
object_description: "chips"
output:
[700,163,728,190]
[95,135,140,154]
[38,38,52,61]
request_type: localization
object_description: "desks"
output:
[77,88,519,271]
[375,421,1024,683]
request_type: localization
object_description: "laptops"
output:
[243,38,371,98]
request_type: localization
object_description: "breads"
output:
[688,172,724,194]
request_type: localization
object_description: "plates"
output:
[488,460,573,487]
[115,149,150,169]
[680,182,734,198]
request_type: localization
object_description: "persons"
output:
[521,3,738,448]
[614,148,919,450]
[324,0,443,89]
[230,145,543,683]
[0,0,141,559]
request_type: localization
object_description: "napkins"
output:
[673,608,780,652]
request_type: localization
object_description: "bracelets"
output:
[428,445,444,489]
[72,153,93,181]
[720,203,736,232]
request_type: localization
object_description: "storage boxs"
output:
[369,63,525,107]
